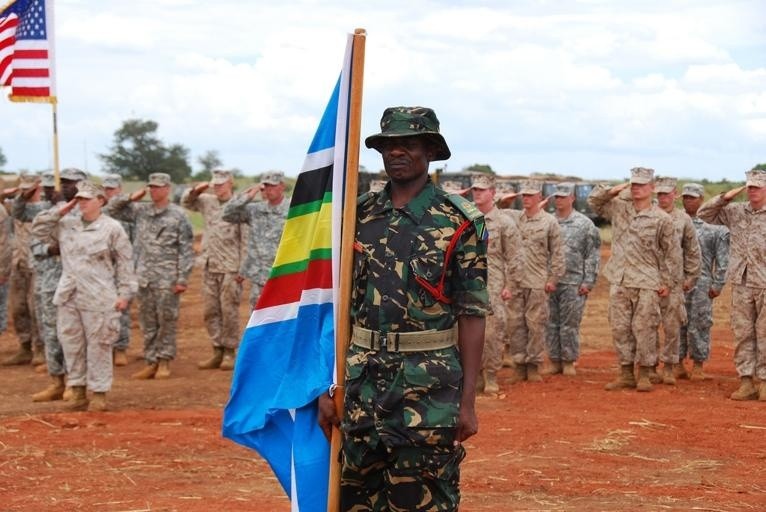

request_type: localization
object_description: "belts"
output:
[350,324,458,354]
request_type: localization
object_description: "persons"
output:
[673,182,728,382]
[585,165,681,392]
[450,172,525,395]
[494,178,565,384]
[316,101,490,511]
[536,180,602,376]
[696,168,766,403]
[0,168,293,413]
[617,176,703,386]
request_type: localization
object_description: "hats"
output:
[58,168,87,182]
[678,183,706,198]
[260,171,286,186]
[75,184,110,203]
[39,172,55,188]
[497,183,515,198]
[369,178,388,194]
[469,174,494,191]
[743,169,766,189]
[550,182,576,197]
[653,177,679,193]
[517,179,544,196]
[147,173,170,188]
[441,180,464,194]
[628,166,655,186]
[18,175,38,191]
[104,173,121,190]
[366,107,450,159]
[209,166,234,186]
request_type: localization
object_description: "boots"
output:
[645,367,663,384]
[503,363,524,384]
[756,378,766,401]
[219,349,234,370]
[673,358,689,379]
[483,372,501,394]
[87,392,108,412]
[62,381,74,400]
[526,361,544,384]
[637,365,651,392]
[31,345,44,364]
[113,348,127,367]
[662,362,677,386]
[197,347,221,371]
[730,376,759,402]
[64,385,88,412]
[475,375,485,393]
[692,359,715,379]
[606,363,636,391]
[562,360,577,377]
[4,345,30,365]
[154,358,171,378]
[29,375,62,401]
[130,358,154,380]
[542,360,560,378]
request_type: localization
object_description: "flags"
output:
[220,29,355,512]
[0,0,57,106]
